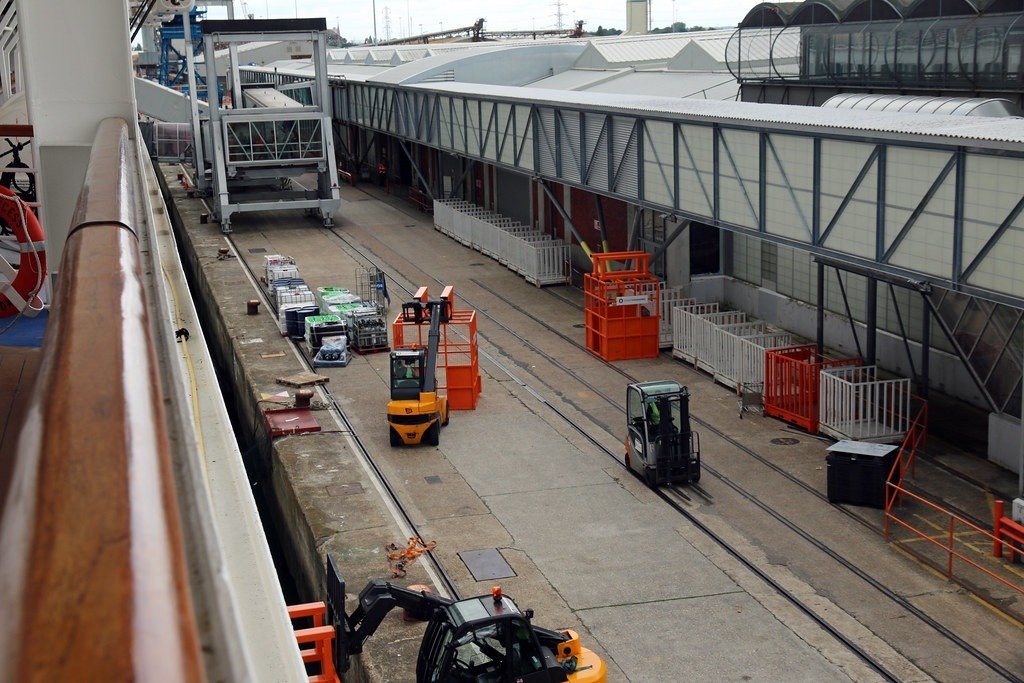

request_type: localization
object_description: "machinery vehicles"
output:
[386,299,452,446]
[626,381,715,502]
[286,551,611,683]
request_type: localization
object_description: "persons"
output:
[646,402,662,427]
